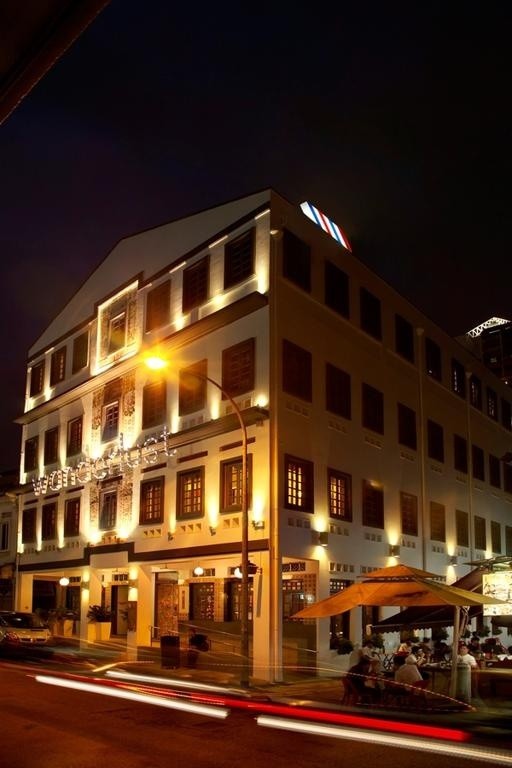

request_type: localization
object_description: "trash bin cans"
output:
[160,636,180,670]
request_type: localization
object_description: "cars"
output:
[1,611,54,658]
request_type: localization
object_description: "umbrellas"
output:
[288,564,511,620]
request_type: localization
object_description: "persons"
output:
[345,634,512,711]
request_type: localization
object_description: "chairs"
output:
[340,677,430,715]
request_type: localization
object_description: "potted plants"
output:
[87,604,118,639]
[48,609,79,636]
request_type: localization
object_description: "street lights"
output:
[149,356,251,687]
